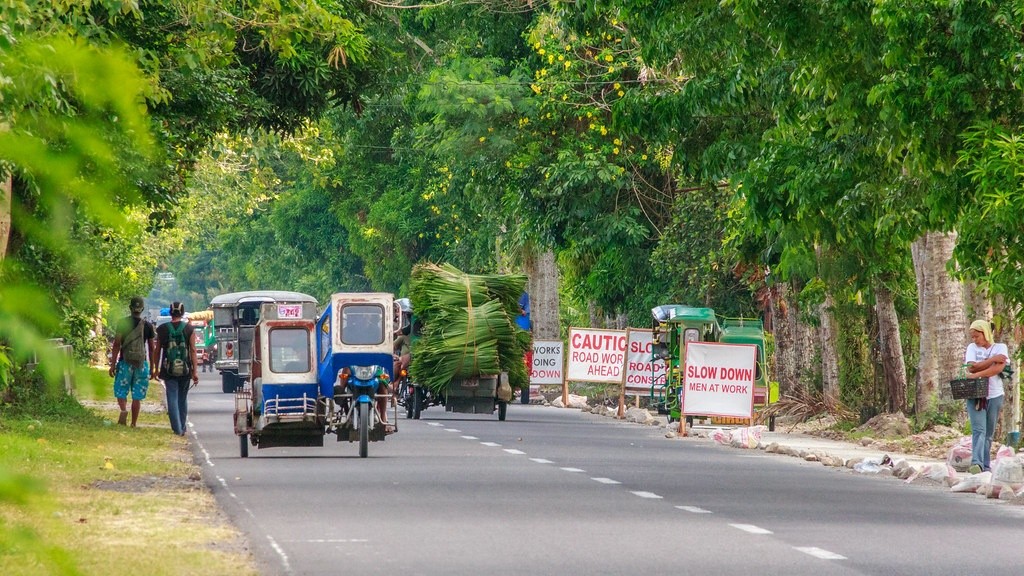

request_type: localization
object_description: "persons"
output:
[334,314,394,433]
[201,349,214,372]
[109,296,154,428]
[154,301,200,437]
[515,290,530,330]
[964,320,1010,473]
[388,309,411,391]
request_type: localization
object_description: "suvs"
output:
[513,289,535,405]
[209,290,319,392]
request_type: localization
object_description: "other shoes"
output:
[970,465,981,473]
[385,426,394,435]
[334,386,346,405]
[117,411,128,426]
[391,395,398,407]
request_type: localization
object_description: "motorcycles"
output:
[391,347,513,420]
[651,302,776,433]
[233,291,399,458]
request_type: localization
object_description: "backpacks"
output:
[122,319,146,367]
[162,322,193,376]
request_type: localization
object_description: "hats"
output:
[169,301,184,317]
[131,297,144,313]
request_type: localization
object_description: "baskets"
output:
[951,365,989,400]
